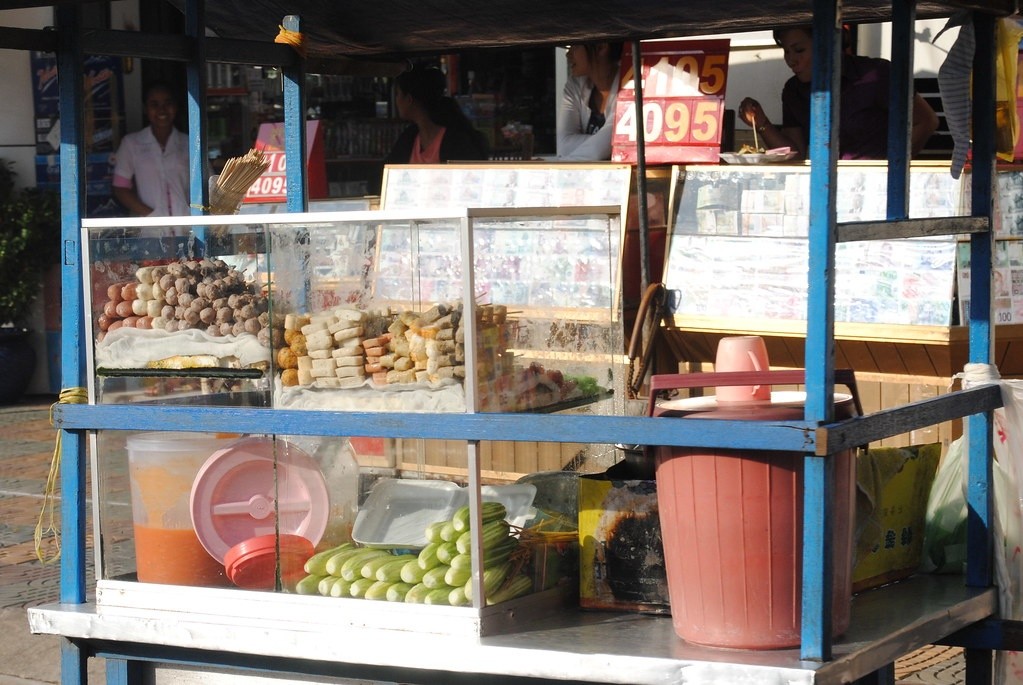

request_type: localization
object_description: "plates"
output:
[717,152,799,164]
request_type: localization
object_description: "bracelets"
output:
[757,118,771,134]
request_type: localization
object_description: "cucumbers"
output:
[295,502,534,607]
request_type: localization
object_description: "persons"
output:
[111,68,191,259]
[737,24,939,160]
[378,68,489,164]
[556,39,624,162]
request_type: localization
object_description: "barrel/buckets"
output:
[124,430,244,589]
[644,367,869,652]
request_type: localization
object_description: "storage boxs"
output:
[575,441,943,617]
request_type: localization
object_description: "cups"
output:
[208,174,247,236]
[714,335,773,402]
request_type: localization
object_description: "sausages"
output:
[95,282,152,340]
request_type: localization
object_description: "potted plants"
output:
[0,157,61,407]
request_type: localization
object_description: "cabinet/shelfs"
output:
[80,203,628,639]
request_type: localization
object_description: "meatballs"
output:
[131,256,285,349]
